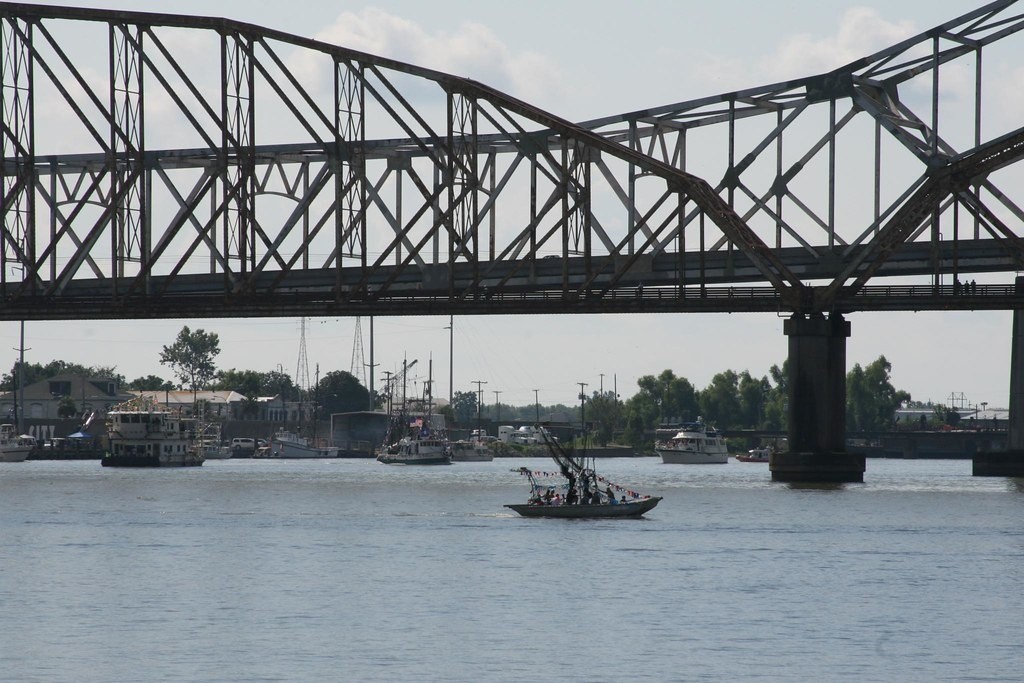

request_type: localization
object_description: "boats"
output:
[735,443,786,463]
[654,416,728,464]
[503,424,664,518]
[270,427,339,459]
[0,424,35,463]
[377,434,494,465]
[101,385,233,468]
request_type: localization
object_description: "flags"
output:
[410,418,422,428]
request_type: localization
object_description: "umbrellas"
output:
[69,432,93,449]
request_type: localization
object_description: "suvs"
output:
[231,438,263,451]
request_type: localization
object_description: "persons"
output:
[536,484,627,504]
[655,438,691,450]
[483,285,490,299]
[956,279,976,295]
[368,286,373,300]
[388,430,447,455]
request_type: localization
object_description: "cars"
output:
[257,439,268,447]
